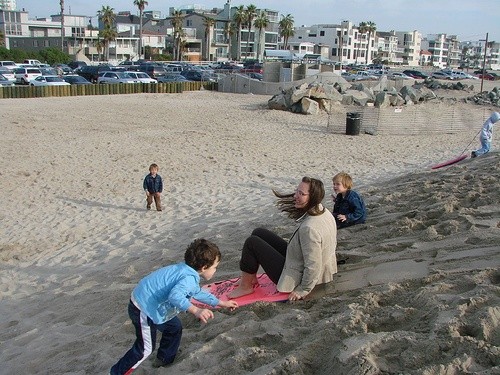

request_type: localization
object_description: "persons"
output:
[332,172,367,230]
[226,177,338,302]
[108,238,239,375]
[471,111,500,159]
[143,163,162,212]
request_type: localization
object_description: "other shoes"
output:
[151,356,167,367]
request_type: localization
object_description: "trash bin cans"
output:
[346,112,360,135]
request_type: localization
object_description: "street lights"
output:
[479,32,488,92]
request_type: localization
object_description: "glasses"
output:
[293,188,310,196]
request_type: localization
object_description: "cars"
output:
[402,69,430,80]
[389,72,414,80]
[441,69,498,81]
[0,58,263,87]
[429,71,453,81]
[339,63,391,82]
